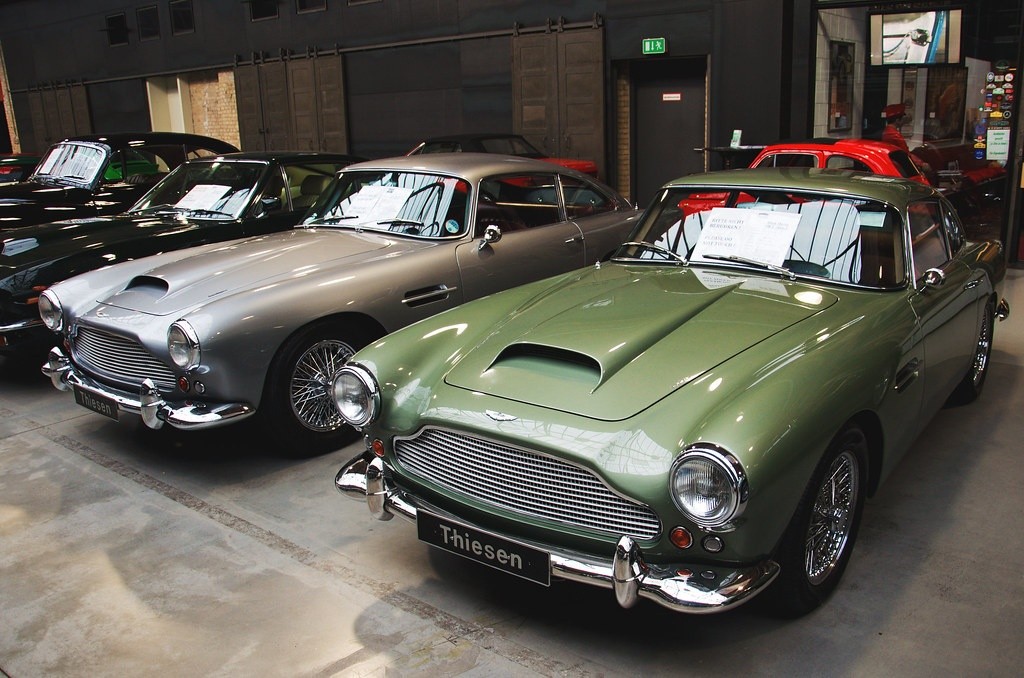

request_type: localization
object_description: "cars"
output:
[331,168,1010,614]
[882,130,1008,188]
[73,144,160,181]
[403,133,602,206]
[0,132,240,231]
[0,153,384,357]
[677,136,941,248]
[38,153,684,454]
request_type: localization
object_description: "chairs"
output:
[125,172,168,185]
[283,174,333,210]
[451,211,533,236]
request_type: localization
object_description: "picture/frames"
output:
[827,40,856,132]
[899,70,918,137]
[922,64,969,145]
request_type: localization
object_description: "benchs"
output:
[926,142,1007,195]
